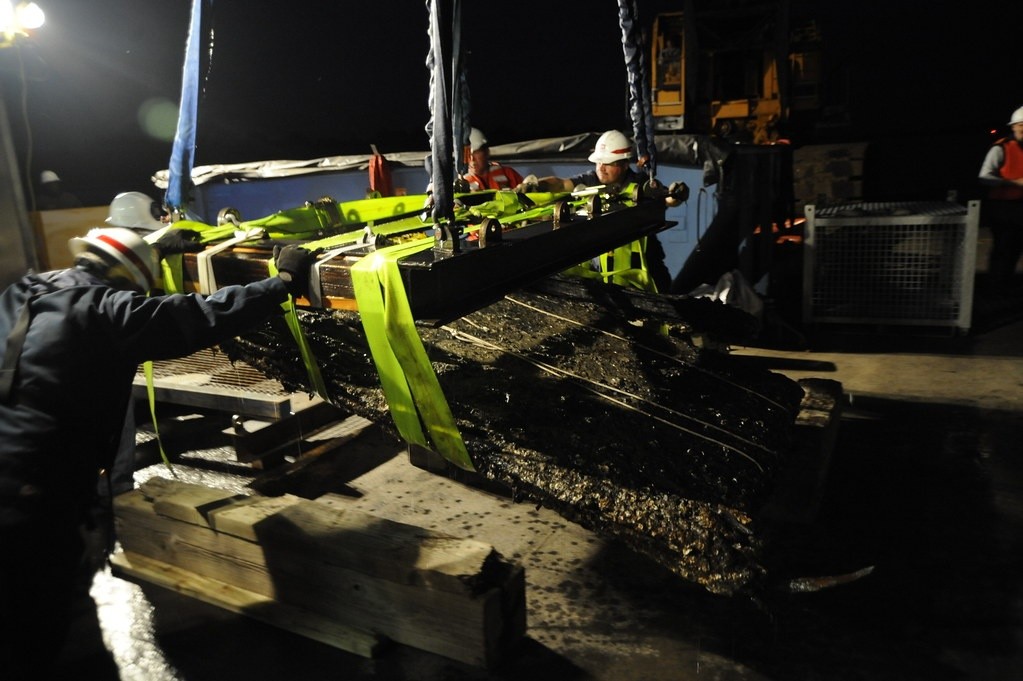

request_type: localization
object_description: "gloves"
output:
[273,244,325,298]
[669,180,690,202]
[513,174,539,193]
[152,229,205,256]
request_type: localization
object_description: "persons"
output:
[463,128,524,190]
[978,106,1023,298]
[516,130,694,345]
[0,192,325,681]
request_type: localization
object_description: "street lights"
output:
[0,3,45,271]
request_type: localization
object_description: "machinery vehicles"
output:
[652,11,822,147]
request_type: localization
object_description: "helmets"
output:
[105,192,171,231]
[67,227,156,294]
[588,128,633,163]
[1007,105,1023,125]
[469,127,487,154]
